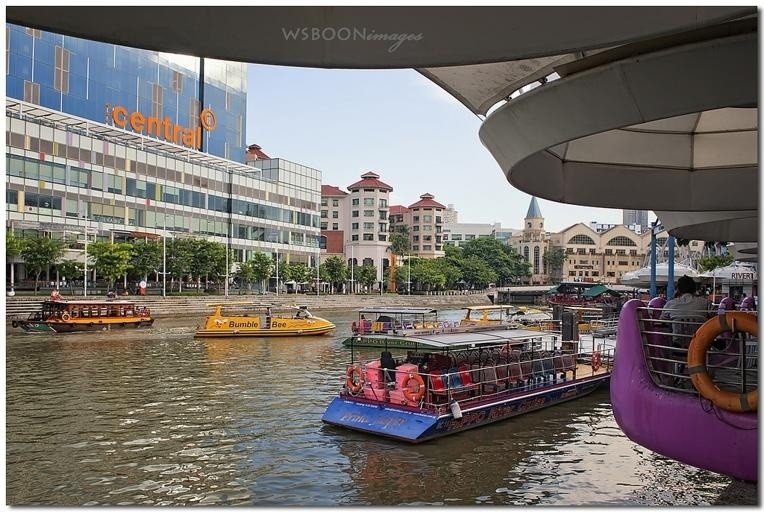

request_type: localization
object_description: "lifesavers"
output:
[740,296,753,312]
[62,314,70,322]
[347,366,365,391]
[688,313,759,412]
[12,320,18,328]
[592,352,601,371]
[402,374,426,401]
[718,297,735,314]
[648,298,666,316]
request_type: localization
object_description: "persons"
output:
[49,287,118,300]
[660,275,721,393]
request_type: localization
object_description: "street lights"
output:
[79,197,91,297]
[223,214,412,297]
[163,212,170,298]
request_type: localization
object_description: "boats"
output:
[460,281,642,334]
[342,306,517,349]
[192,301,337,337]
[12,297,156,332]
[322,336,617,445]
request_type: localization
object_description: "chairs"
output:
[401,348,581,403]
